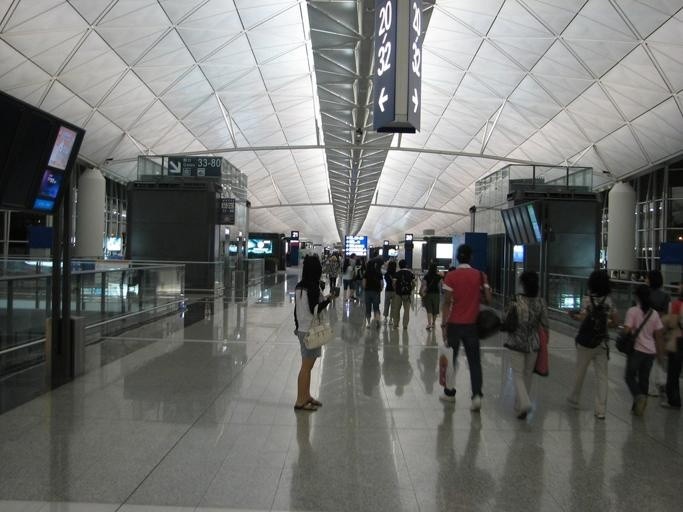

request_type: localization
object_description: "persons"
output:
[564,266,619,425]
[501,268,552,415]
[292,253,333,413]
[436,242,494,411]
[637,268,670,317]
[658,275,682,412]
[614,282,665,422]
[319,247,445,330]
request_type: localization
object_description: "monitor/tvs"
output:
[512,244,524,263]
[434,242,452,259]
[107,236,121,251]
[248,239,273,255]
[378,249,383,255]
[388,248,398,257]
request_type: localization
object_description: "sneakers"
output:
[661,401,681,410]
[513,405,533,420]
[383,319,393,325]
[393,322,398,330]
[631,394,648,417]
[648,384,667,398]
[593,409,606,419]
[565,397,580,409]
[365,320,379,329]
[425,324,436,329]
[403,323,407,329]
[439,393,456,403]
[469,393,482,412]
[342,296,360,302]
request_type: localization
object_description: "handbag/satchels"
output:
[501,294,519,333]
[477,308,501,340]
[391,278,397,290]
[533,321,549,378]
[615,331,634,354]
[664,301,676,352]
[438,339,456,391]
[302,303,337,350]
[419,278,428,297]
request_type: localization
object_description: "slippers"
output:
[294,396,323,411]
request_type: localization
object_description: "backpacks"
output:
[396,270,412,295]
[574,294,611,348]
